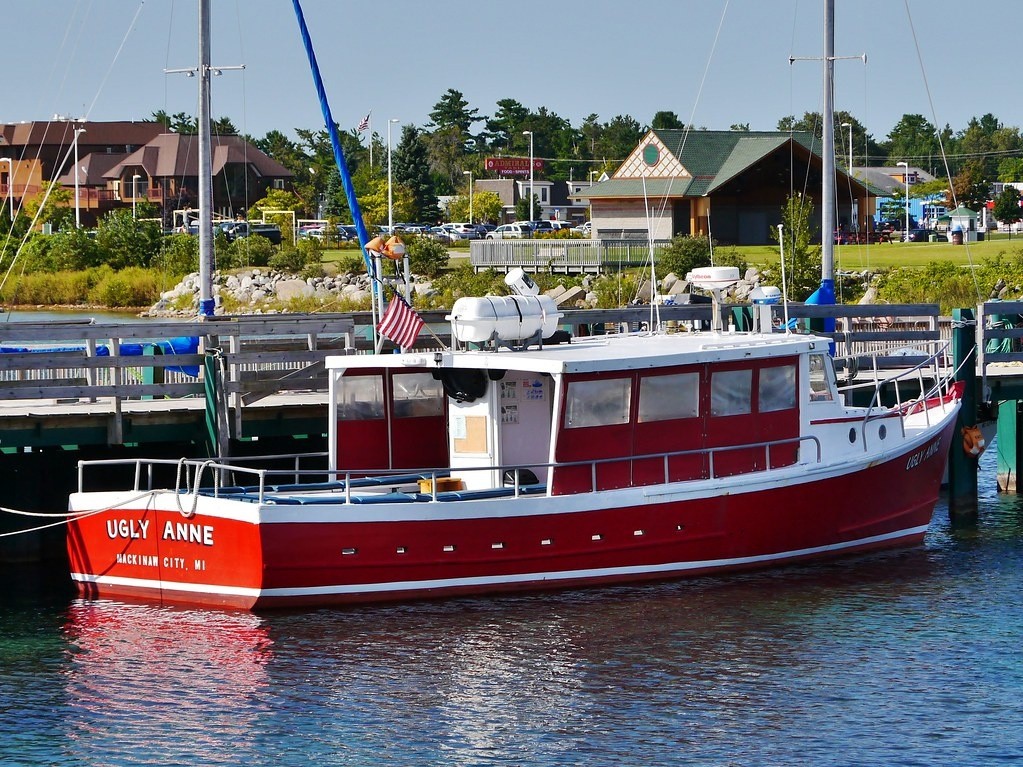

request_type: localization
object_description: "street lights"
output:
[897,161,909,242]
[463,170,472,225]
[74,129,86,229]
[0,157,14,222]
[523,130,533,222]
[133,175,141,222]
[842,123,853,176]
[387,119,399,236]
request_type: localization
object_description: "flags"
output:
[375,292,424,351]
[357,115,369,131]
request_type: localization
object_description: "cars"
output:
[909,228,939,242]
[163,219,591,246]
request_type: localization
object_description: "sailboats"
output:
[47,0,980,616]
